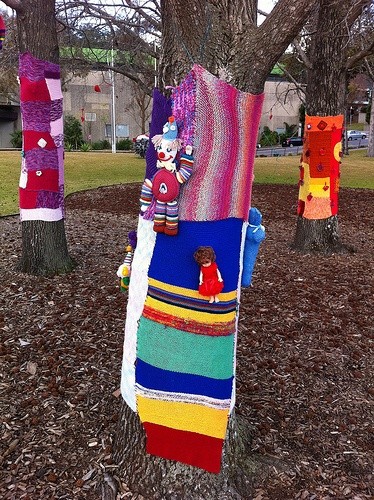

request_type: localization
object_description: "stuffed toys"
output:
[140,117,193,234]
[242,208,266,287]
[117,231,137,295]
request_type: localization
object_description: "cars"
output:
[255,143,262,150]
[343,129,368,141]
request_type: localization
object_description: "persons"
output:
[282,138,293,146]
[193,247,223,304]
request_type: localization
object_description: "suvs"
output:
[281,136,304,148]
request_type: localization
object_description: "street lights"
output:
[109,29,139,155]
